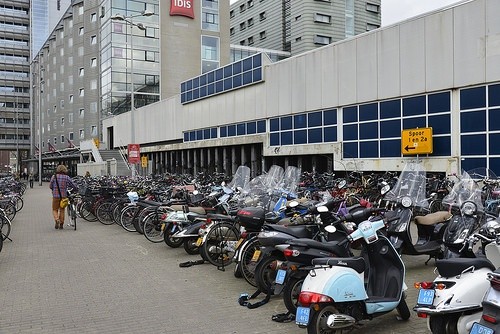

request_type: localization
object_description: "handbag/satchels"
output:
[60,198,69,208]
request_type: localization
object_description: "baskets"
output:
[79,186,86,195]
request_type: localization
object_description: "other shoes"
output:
[55,220,59,229]
[59,223,63,229]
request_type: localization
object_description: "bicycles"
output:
[0,172,28,250]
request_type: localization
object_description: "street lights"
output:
[114,14,147,175]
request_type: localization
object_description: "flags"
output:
[49,143,55,152]
[67,140,72,147]
[35,146,39,153]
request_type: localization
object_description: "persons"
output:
[49,165,79,229]
[23,172,34,188]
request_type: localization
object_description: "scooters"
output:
[64,159,500,334]
